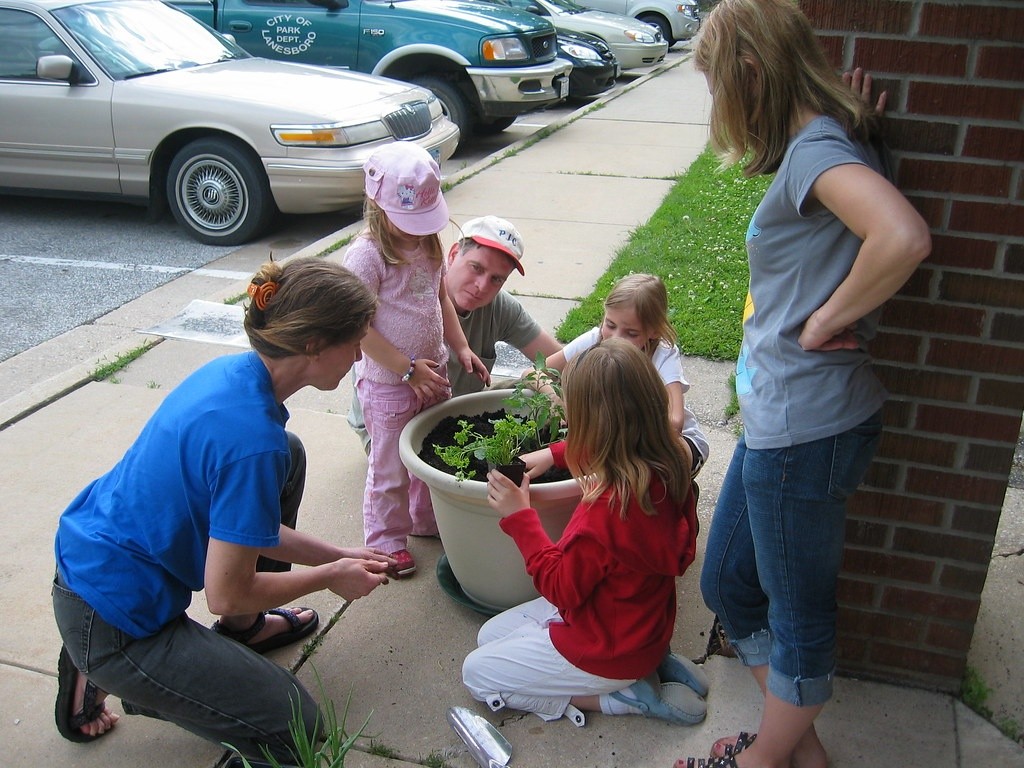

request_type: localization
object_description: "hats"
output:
[459,214,526,277]
[362,141,450,238]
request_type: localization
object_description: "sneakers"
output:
[608,650,711,724]
[388,550,416,577]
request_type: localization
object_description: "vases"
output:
[398,389,597,612]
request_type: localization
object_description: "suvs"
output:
[83,0,575,161]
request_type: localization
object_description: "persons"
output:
[520,274,710,540]
[51,258,398,765]
[347,215,564,457]
[672,0,932,768]
[341,141,491,576]
[462,337,708,727]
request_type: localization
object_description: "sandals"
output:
[205,605,321,651]
[54,641,119,746]
[672,730,758,768]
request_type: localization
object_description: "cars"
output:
[571,0,702,50]
[480,0,670,76]
[554,26,622,102]
[0,0,462,246]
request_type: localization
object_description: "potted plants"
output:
[432,414,526,486]
[502,350,567,450]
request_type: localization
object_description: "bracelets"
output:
[400,356,416,382]
